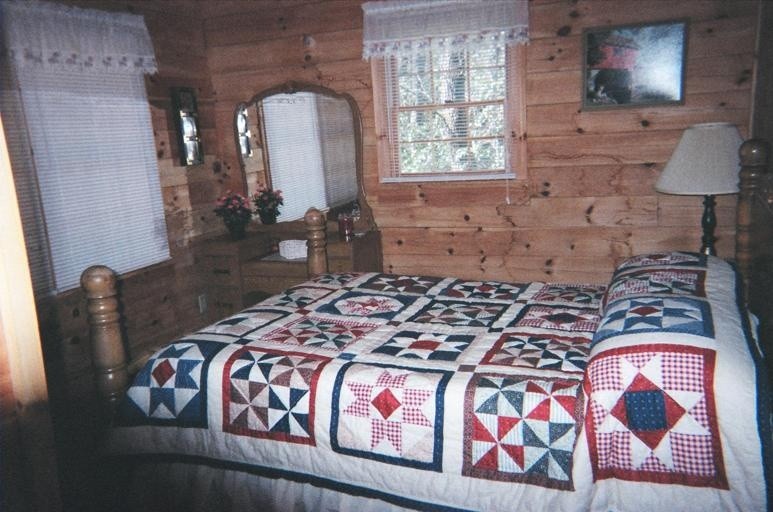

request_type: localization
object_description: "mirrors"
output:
[234,81,364,228]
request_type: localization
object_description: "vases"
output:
[259,211,276,225]
[227,222,245,238]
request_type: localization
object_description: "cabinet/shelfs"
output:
[207,229,381,323]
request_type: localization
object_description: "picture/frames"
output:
[581,17,688,112]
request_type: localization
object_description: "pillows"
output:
[585,252,764,401]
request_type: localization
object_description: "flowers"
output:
[212,190,252,226]
[250,185,284,214]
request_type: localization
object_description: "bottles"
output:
[338,236,361,244]
[337,203,364,236]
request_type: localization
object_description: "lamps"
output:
[653,122,753,256]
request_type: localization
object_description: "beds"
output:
[79,139,772,509]
